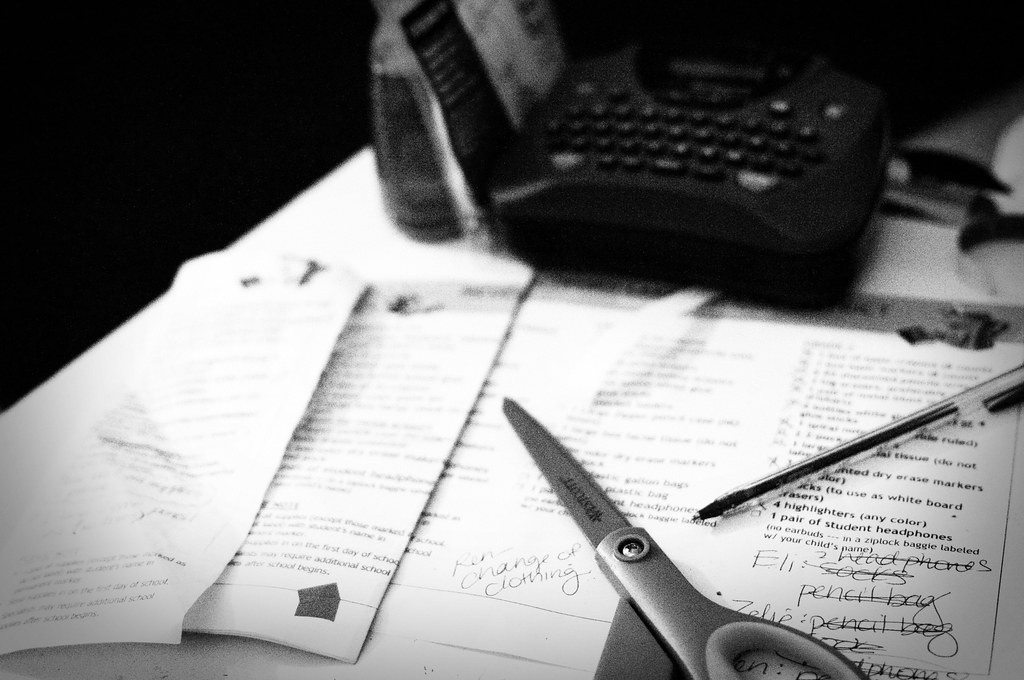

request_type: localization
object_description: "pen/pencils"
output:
[692,363,1024,526]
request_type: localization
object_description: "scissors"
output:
[500,396,875,680]
